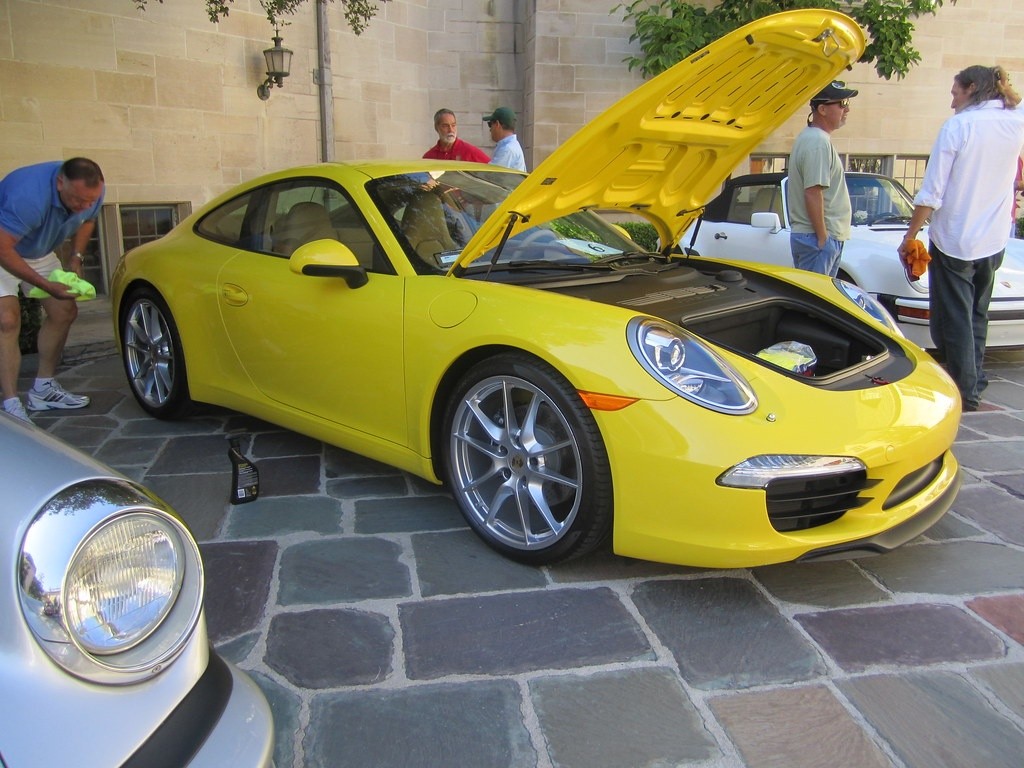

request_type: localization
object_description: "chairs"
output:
[752,188,784,228]
[400,192,461,272]
[276,201,341,258]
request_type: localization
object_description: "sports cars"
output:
[113,6,964,571]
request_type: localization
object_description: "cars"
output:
[1,407,283,768]
[653,172,1024,356]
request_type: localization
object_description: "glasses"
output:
[824,99,850,108]
[487,121,496,128]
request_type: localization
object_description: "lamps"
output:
[256,22,295,100]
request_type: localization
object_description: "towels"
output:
[899,235,933,283]
[26,269,97,302]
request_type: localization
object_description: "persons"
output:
[788,79,858,278]
[0,157,106,426]
[1010,156,1024,238]
[482,108,527,172]
[422,109,490,163]
[897,65,1024,411]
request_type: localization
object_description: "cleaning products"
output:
[228,436,261,505]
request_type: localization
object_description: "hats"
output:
[809,79,859,100]
[482,107,517,128]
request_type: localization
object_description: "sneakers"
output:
[27,380,90,410]
[3,397,36,427]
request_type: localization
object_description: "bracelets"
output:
[71,252,85,265]
